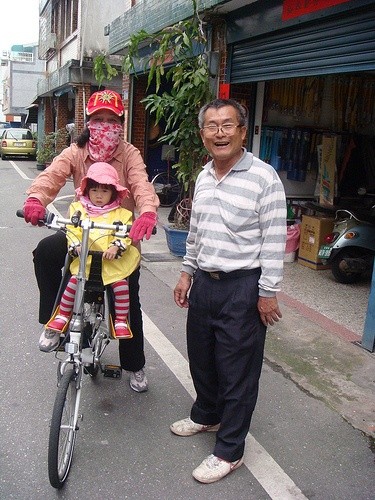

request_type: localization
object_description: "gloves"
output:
[23,197,46,227]
[129,212,157,243]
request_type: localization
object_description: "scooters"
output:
[317,194,375,284]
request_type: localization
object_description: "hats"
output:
[81,162,127,200]
[85,90,124,117]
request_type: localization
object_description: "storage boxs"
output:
[319,134,368,208]
[297,215,330,271]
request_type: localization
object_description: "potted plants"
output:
[32,132,52,170]
[92,0,216,257]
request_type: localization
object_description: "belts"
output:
[209,268,260,281]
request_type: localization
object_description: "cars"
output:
[0,128,37,161]
[0,121,22,139]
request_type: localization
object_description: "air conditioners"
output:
[46,33,56,53]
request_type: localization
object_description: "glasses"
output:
[203,123,245,138]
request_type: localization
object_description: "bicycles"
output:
[150,159,184,207]
[14,209,156,490]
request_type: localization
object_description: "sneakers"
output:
[128,369,147,392]
[192,452,244,484]
[39,328,59,352]
[170,417,222,437]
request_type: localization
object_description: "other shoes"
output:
[114,320,130,336]
[49,315,70,330]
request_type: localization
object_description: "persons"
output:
[169,97,288,481]
[44,162,141,340]
[23,90,160,393]
